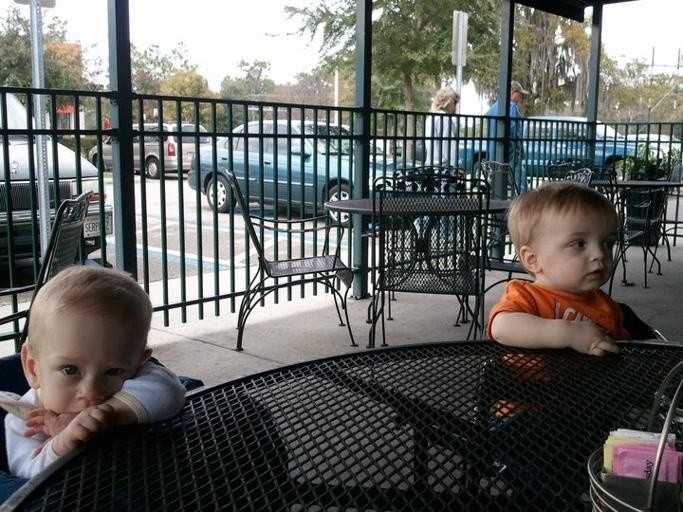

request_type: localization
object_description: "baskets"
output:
[586,361,681,511]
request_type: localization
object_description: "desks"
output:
[0,340,683,511]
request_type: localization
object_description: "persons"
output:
[413,86,461,243]
[477,183,631,470]
[479,80,530,194]
[0,263,183,479]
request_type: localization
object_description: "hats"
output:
[511,80,532,95]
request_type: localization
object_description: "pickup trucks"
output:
[456,115,666,179]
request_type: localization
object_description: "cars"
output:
[187,117,427,228]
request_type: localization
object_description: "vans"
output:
[87,122,215,179]
[0,86,114,268]
